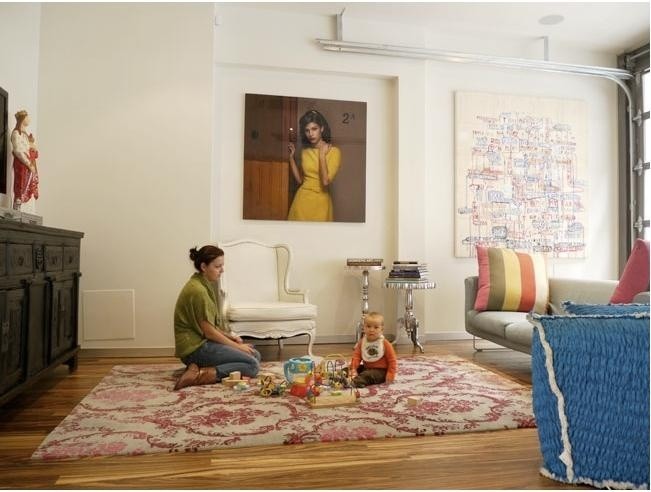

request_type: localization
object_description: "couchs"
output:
[464,275,650,359]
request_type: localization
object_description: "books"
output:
[385,260,429,283]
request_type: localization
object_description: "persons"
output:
[10,110,36,212]
[287,110,341,221]
[334,312,397,388]
[174,244,262,390]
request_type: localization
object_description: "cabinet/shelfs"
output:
[0,219,85,409]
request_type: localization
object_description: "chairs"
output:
[529,299,650,490]
[213,237,318,361]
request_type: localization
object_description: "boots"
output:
[171,362,218,391]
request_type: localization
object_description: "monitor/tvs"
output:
[0,86,12,209]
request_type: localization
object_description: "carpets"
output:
[29,352,536,461]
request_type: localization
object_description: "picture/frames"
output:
[0,85,9,195]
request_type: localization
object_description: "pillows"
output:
[473,246,549,316]
[609,238,650,304]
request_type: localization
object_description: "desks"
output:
[382,276,436,355]
[345,264,385,352]
